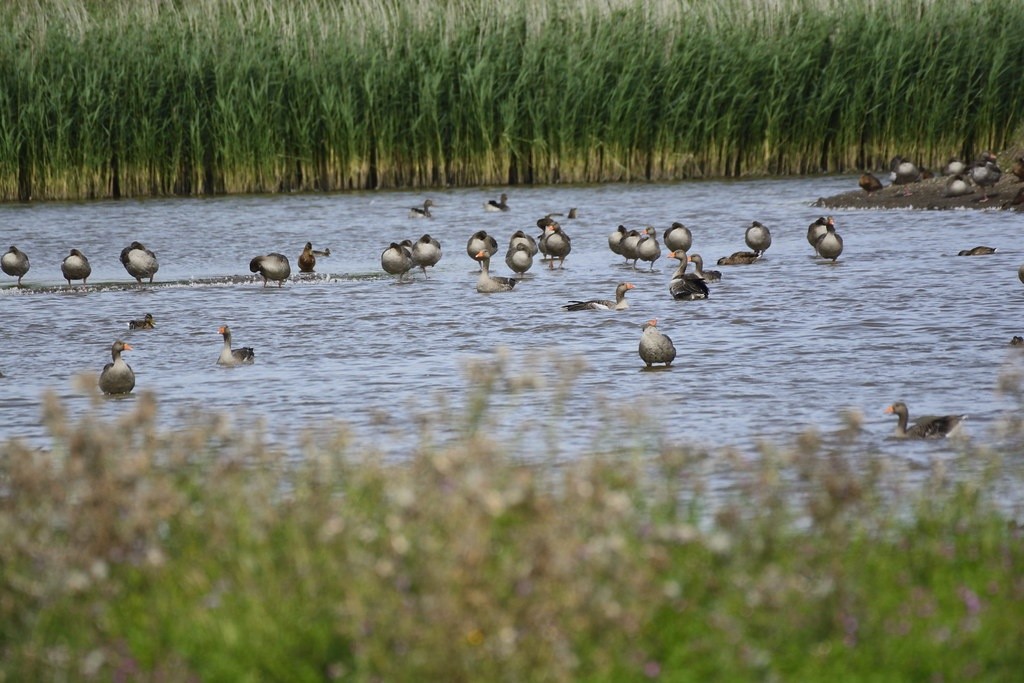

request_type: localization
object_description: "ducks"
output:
[0,150,1024,444]
